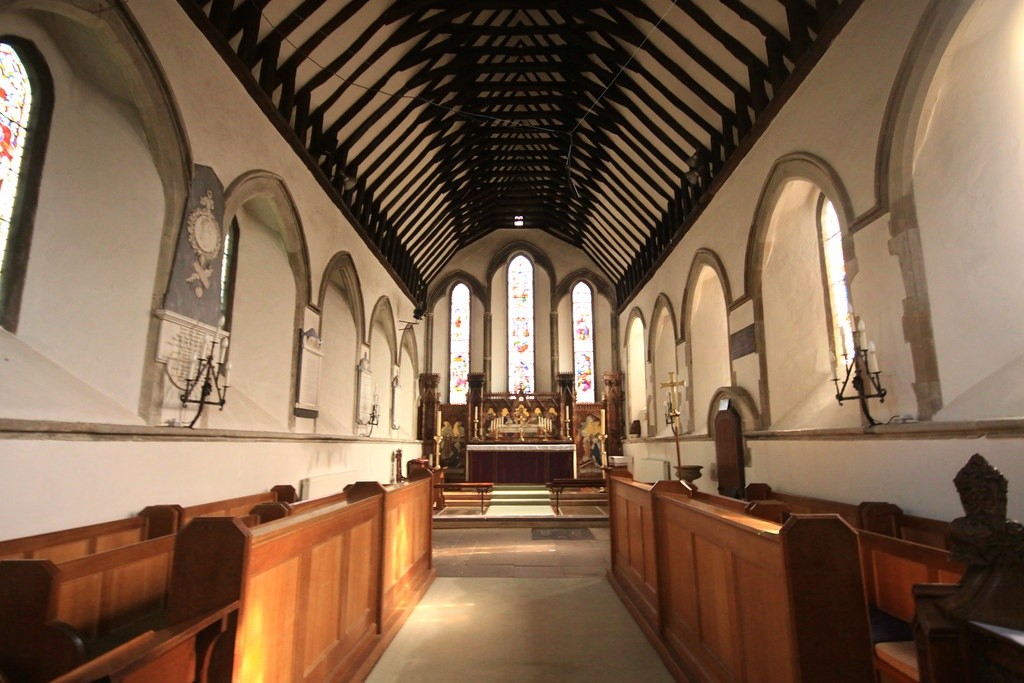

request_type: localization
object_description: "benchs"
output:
[432,479,494,510]
[546,478,608,512]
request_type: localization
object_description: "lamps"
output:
[180,339,231,430]
[828,312,887,429]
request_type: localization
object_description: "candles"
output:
[600,409,605,435]
[436,410,442,437]
[565,405,570,420]
[428,453,433,467]
[474,406,478,421]
